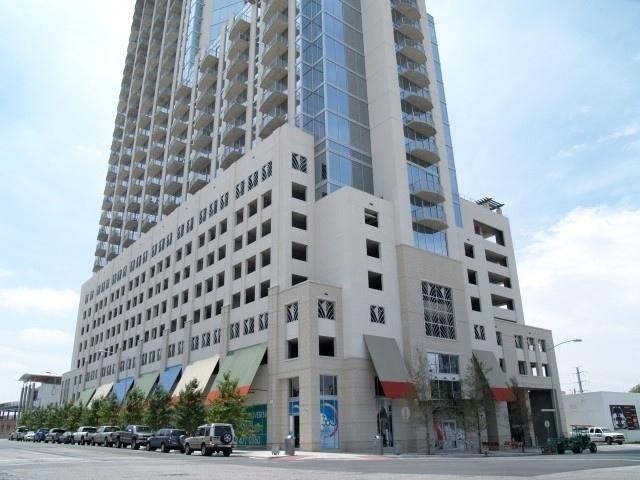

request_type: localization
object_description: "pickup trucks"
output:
[571,426,625,445]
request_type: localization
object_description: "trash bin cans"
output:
[285,435,296,455]
[375,436,383,455]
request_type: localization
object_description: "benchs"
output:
[482,442,499,451]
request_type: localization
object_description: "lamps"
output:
[46,372,62,374]
[549,339,582,350]
[528,390,530,393]
[98,350,113,353]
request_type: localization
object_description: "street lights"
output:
[97,349,127,427]
[45,372,70,408]
[546,338,582,438]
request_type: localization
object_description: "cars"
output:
[7,422,238,456]
